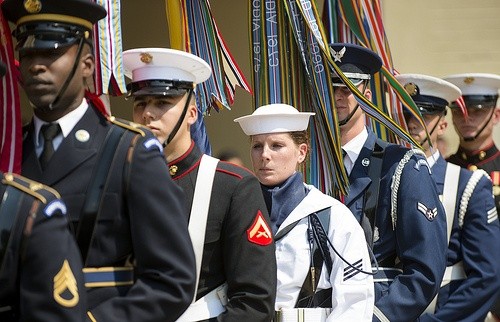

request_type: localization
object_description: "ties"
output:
[38,124,62,171]
[340,149,349,178]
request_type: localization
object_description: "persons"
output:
[329,43,447,322]
[0,0,196,322]
[233,103,374,322]
[119,47,277,322]
[394,74,500,322]
[0,175,91,322]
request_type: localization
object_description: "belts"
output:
[175,282,229,322]
[82,265,135,286]
[442,262,466,280]
[372,267,404,283]
[269,304,333,322]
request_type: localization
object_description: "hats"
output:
[443,73,500,109]
[2,0,107,50]
[121,48,212,100]
[328,43,383,86]
[394,74,463,115]
[234,104,316,136]
